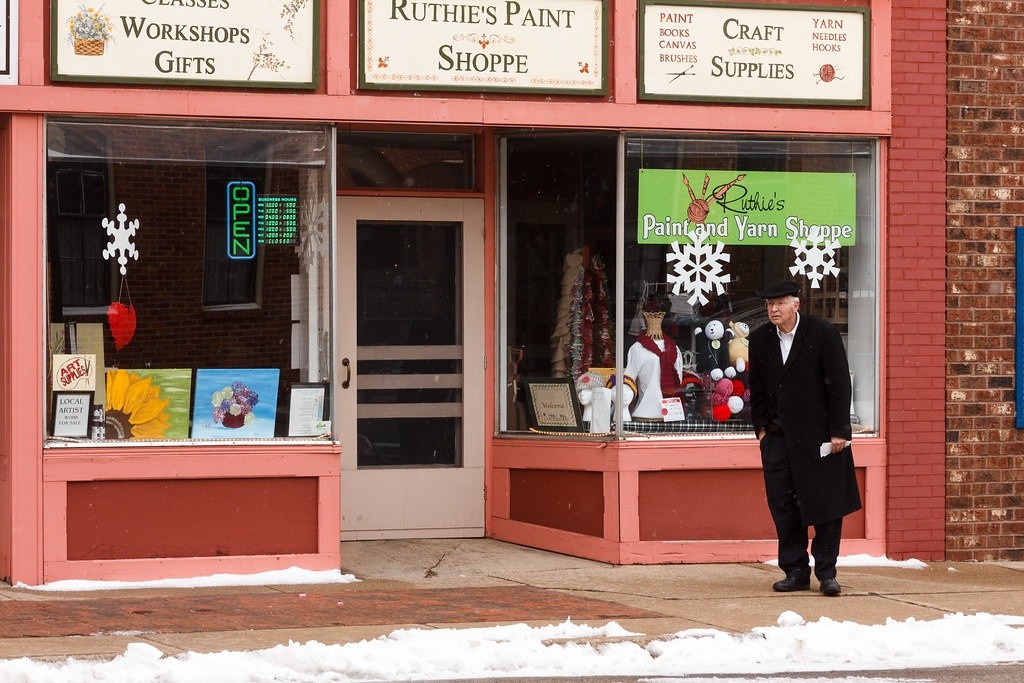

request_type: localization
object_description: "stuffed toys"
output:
[695,317,734,372]
[724,319,752,369]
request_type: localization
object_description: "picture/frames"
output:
[284,382,330,436]
[193,369,281,438]
[50,390,95,438]
[105,367,191,438]
[523,378,583,433]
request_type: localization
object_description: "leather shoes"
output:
[819,578,842,595]
[773,570,810,591]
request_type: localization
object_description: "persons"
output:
[747,276,865,595]
[602,374,637,423]
[575,372,606,422]
[624,302,686,421]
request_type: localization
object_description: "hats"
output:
[761,281,801,299]
